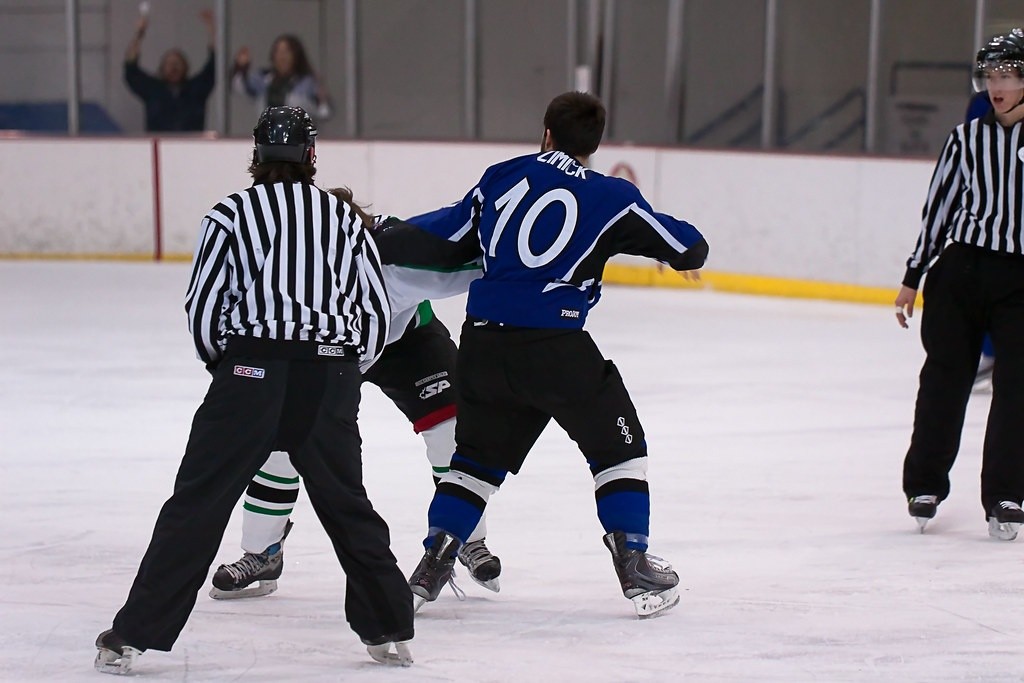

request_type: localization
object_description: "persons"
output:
[891,28,1024,539]
[96,107,416,675]
[230,34,331,132]
[368,94,709,619]
[208,186,503,598]
[123,0,217,135]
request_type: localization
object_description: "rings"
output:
[895,305,904,314]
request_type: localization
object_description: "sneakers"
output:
[93,629,146,674]
[971,352,996,394]
[458,537,502,593]
[208,518,294,600]
[407,526,463,615]
[603,531,681,618]
[988,499,1024,540]
[909,495,941,534]
[360,624,414,668]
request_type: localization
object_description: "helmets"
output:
[253,105,318,166]
[972,27,1024,93]
